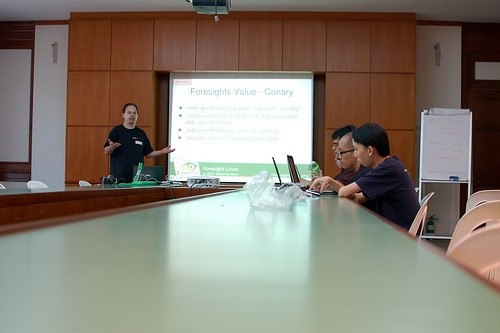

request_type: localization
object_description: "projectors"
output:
[187,176,221,188]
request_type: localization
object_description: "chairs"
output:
[407,191,435,241]
[27,180,49,189]
[447,223,499,288]
[446,199,499,253]
[465,189,500,227]
[79,180,92,187]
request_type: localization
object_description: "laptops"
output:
[271,155,316,192]
[133,166,164,181]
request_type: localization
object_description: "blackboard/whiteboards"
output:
[419,107,471,183]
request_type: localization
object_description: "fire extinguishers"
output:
[427,214,438,240]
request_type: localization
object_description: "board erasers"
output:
[449,176,459,181]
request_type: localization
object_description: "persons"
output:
[298,125,356,188]
[335,130,376,213]
[103,103,175,184]
[309,122,422,237]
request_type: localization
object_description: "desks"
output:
[0,184,500,333]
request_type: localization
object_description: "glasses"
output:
[338,149,355,156]
